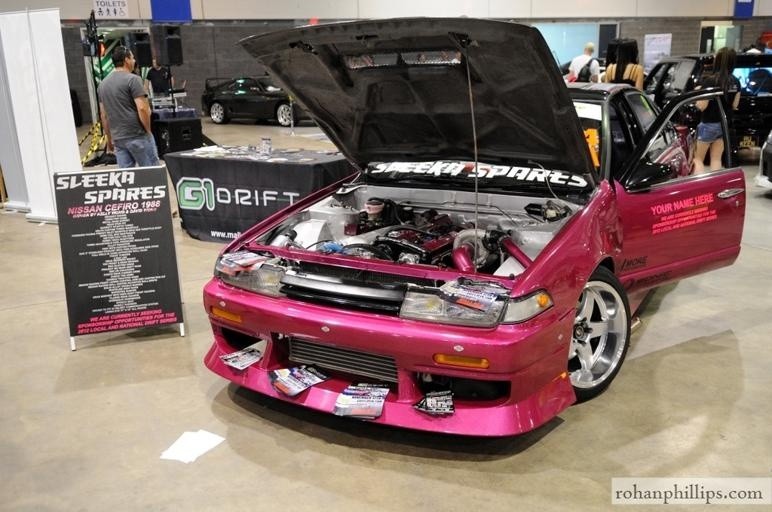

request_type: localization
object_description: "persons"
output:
[568,40,600,83]
[744,44,762,55]
[601,38,623,82]
[143,56,174,97]
[604,39,643,92]
[95,43,178,221]
[692,44,742,175]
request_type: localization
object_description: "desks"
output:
[163,145,354,244]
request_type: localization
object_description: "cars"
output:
[200,75,312,128]
[201,15,748,441]
[642,52,772,168]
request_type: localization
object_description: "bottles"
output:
[260,137,271,156]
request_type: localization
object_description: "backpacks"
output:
[578,57,595,82]
